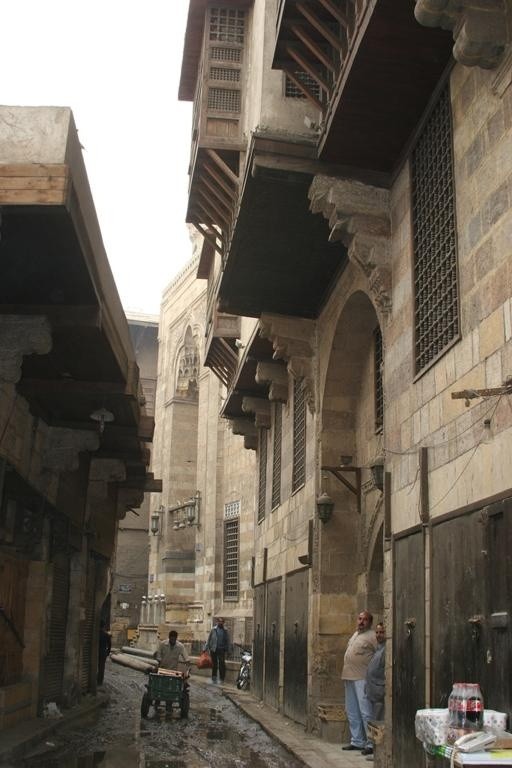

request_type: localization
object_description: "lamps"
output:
[316,469,336,524]
[90,396,114,433]
[184,490,201,526]
[480,395,494,445]
[150,505,164,537]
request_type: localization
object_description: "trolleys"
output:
[140,659,194,718]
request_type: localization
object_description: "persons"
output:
[341,608,385,761]
[99,616,233,712]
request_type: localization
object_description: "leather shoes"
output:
[342,744,374,761]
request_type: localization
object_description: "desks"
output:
[423,744,512,768]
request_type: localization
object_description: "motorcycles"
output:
[233,641,252,688]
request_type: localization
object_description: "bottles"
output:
[449,682,483,730]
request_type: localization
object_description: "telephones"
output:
[454,731,497,753]
